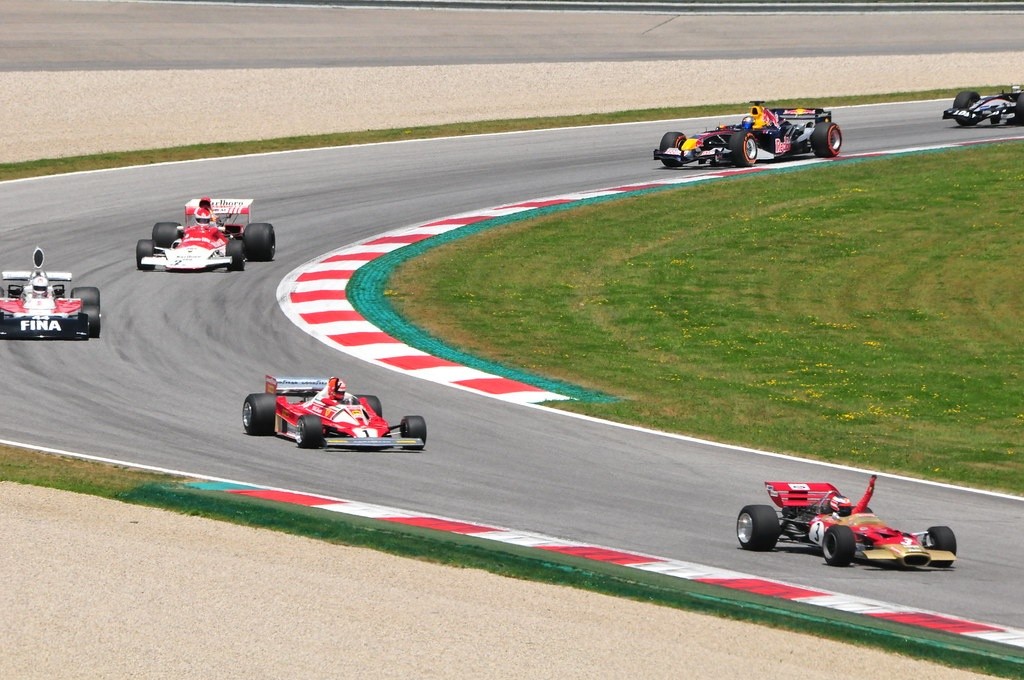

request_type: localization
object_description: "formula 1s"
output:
[242,375,427,453]
[942,86,1024,127]
[136,198,276,273]
[736,475,956,570]
[653,100,843,169]
[1,246,102,341]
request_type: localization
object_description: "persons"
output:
[328,377,346,401]
[742,116,755,129]
[830,475,877,516]
[32,277,49,299]
[194,208,211,227]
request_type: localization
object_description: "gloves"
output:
[869,475,877,488]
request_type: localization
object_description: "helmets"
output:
[742,116,756,129]
[829,495,851,512]
[31,277,49,294]
[194,206,211,227]
[326,378,347,400]
